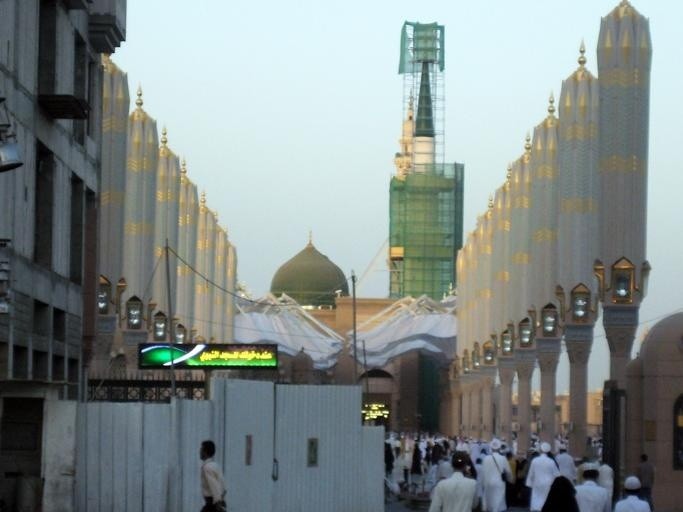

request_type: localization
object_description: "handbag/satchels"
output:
[500,472,507,481]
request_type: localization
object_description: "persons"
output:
[199,440,228,512]
[384,428,657,512]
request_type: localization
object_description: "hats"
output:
[584,463,597,470]
[559,444,566,451]
[625,476,642,490]
[490,439,501,449]
[540,443,551,453]
[453,451,467,461]
